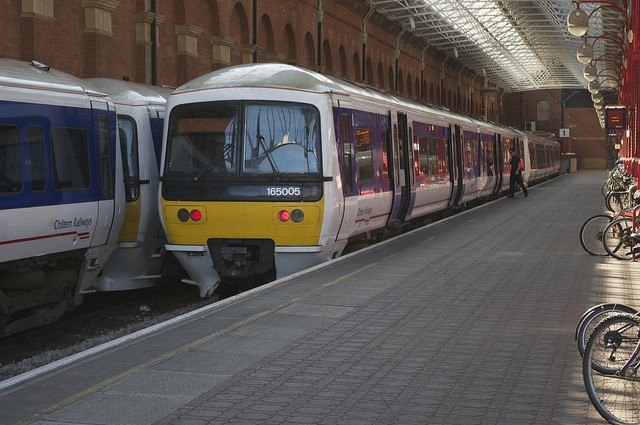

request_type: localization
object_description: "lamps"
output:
[567,1,630,36]
[588,68,620,95]
[592,88,621,131]
[583,52,623,81]
[576,34,627,65]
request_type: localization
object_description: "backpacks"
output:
[520,158,525,170]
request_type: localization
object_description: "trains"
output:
[159,61,562,296]
[0,59,173,339]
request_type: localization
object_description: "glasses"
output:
[509,151,514,153]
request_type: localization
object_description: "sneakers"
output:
[507,193,514,197]
[525,189,528,197]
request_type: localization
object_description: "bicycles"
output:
[580,192,639,256]
[602,163,635,212]
[582,313,640,425]
[575,303,640,374]
[602,205,640,260]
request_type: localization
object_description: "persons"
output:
[464,142,474,180]
[508,148,528,198]
